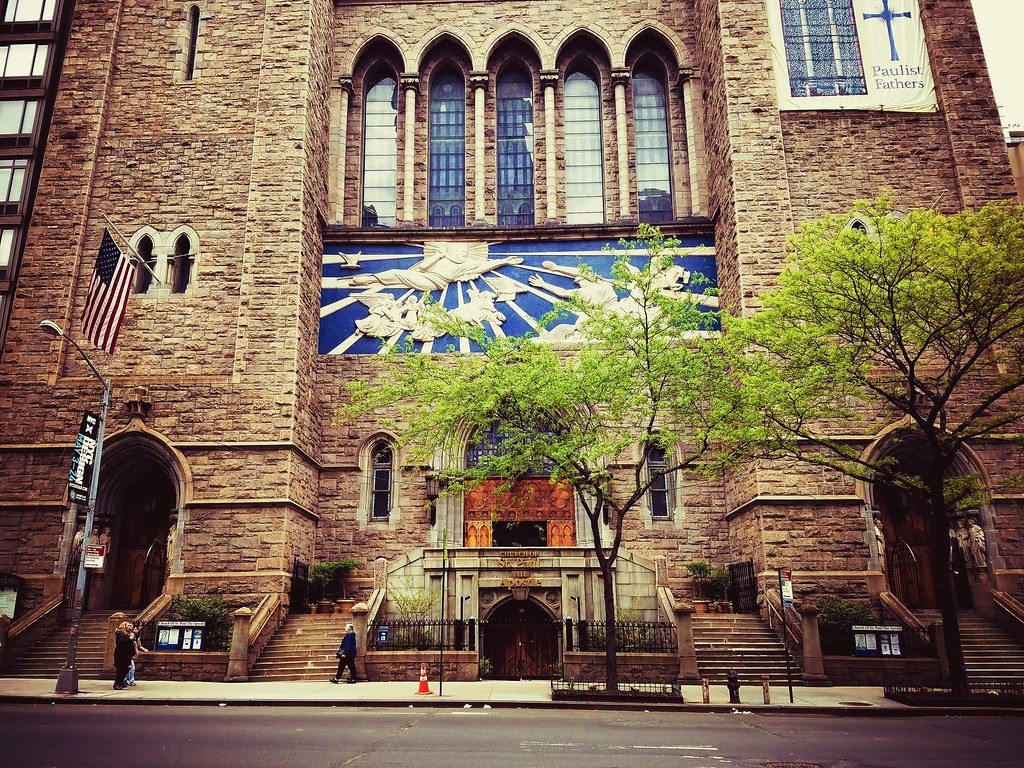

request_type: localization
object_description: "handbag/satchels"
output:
[336,650,349,661]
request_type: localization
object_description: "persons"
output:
[948,517,987,567]
[874,518,885,571]
[354,301,394,335]
[350,242,524,291]
[400,295,420,330]
[528,257,683,330]
[124,626,149,686]
[329,624,357,684]
[73,524,110,573]
[113,622,135,690]
[167,524,177,565]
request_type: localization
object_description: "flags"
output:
[80,227,135,355]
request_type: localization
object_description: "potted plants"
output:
[556,661,564,682]
[307,559,358,614]
[479,655,493,679]
[685,559,734,614]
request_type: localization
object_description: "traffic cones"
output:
[415,663,433,695]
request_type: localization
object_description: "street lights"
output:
[41,318,113,697]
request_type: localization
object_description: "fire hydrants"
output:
[726,667,743,704]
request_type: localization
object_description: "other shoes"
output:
[129,681,137,686]
[347,679,356,683]
[123,680,129,685]
[114,684,122,689]
[120,682,127,688]
[329,679,338,684]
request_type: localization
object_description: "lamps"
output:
[599,472,613,525]
[424,474,444,526]
